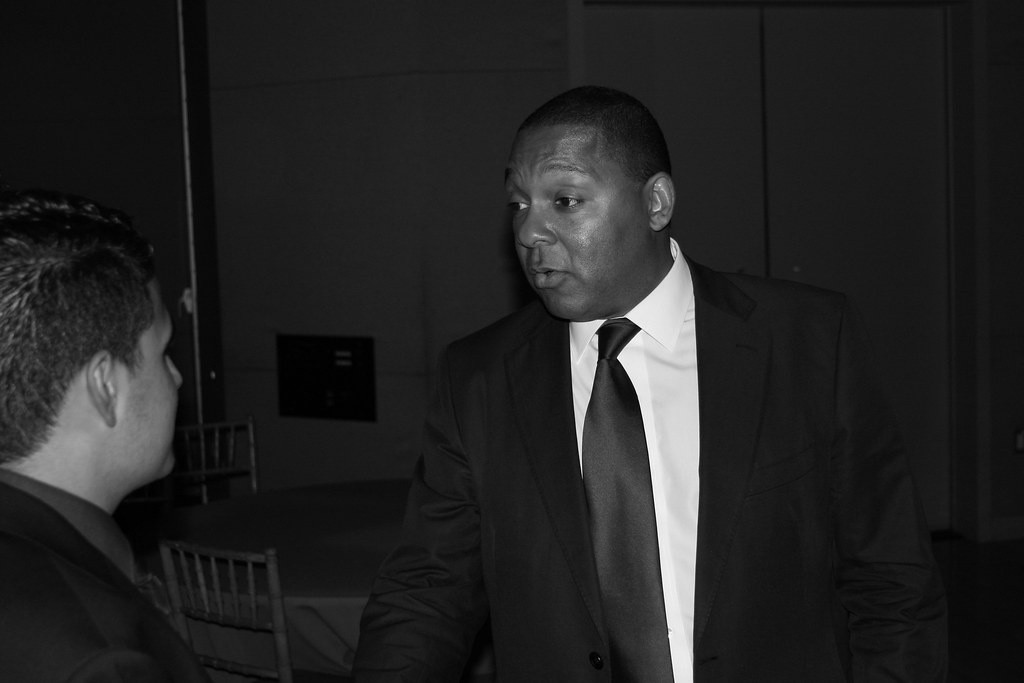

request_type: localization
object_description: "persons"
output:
[0,198,210,683]
[349,86,937,683]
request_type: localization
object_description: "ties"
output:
[134,555,180,638]
[582,318,674,683]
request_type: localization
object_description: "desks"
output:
[143,478,499,683]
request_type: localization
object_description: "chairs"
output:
[165,414,259,511]
[158,536,355,683]
